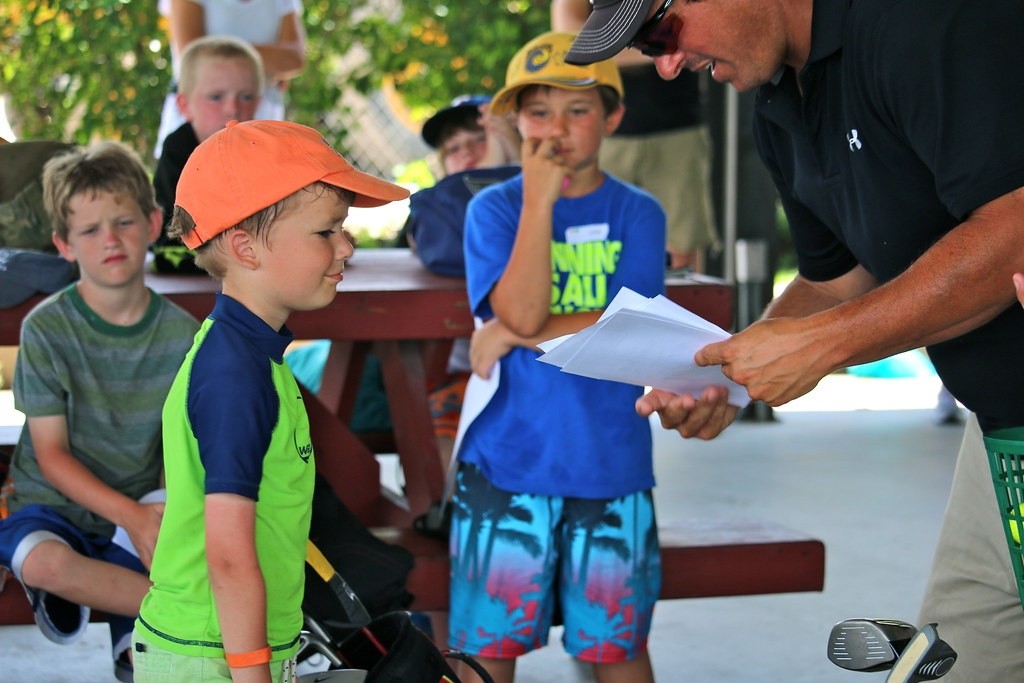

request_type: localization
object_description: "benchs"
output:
[0,241,822,601]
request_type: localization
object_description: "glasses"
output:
[626,0,683,56]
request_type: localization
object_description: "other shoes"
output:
[934,390,963,424]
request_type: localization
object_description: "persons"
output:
[450,26,673,682]
[1,141,206,681]
[132,115,414,683]
[145,34,261,273]
[394,89,520,503]
[150,2,305,159]
[552,1,720,274]
[565,1,1023,683]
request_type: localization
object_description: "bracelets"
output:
[226,644,273,668]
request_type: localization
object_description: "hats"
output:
[489,30,625,117]
[564,0,655,66]
[421,92,492,147]
[173,117,409,251]
